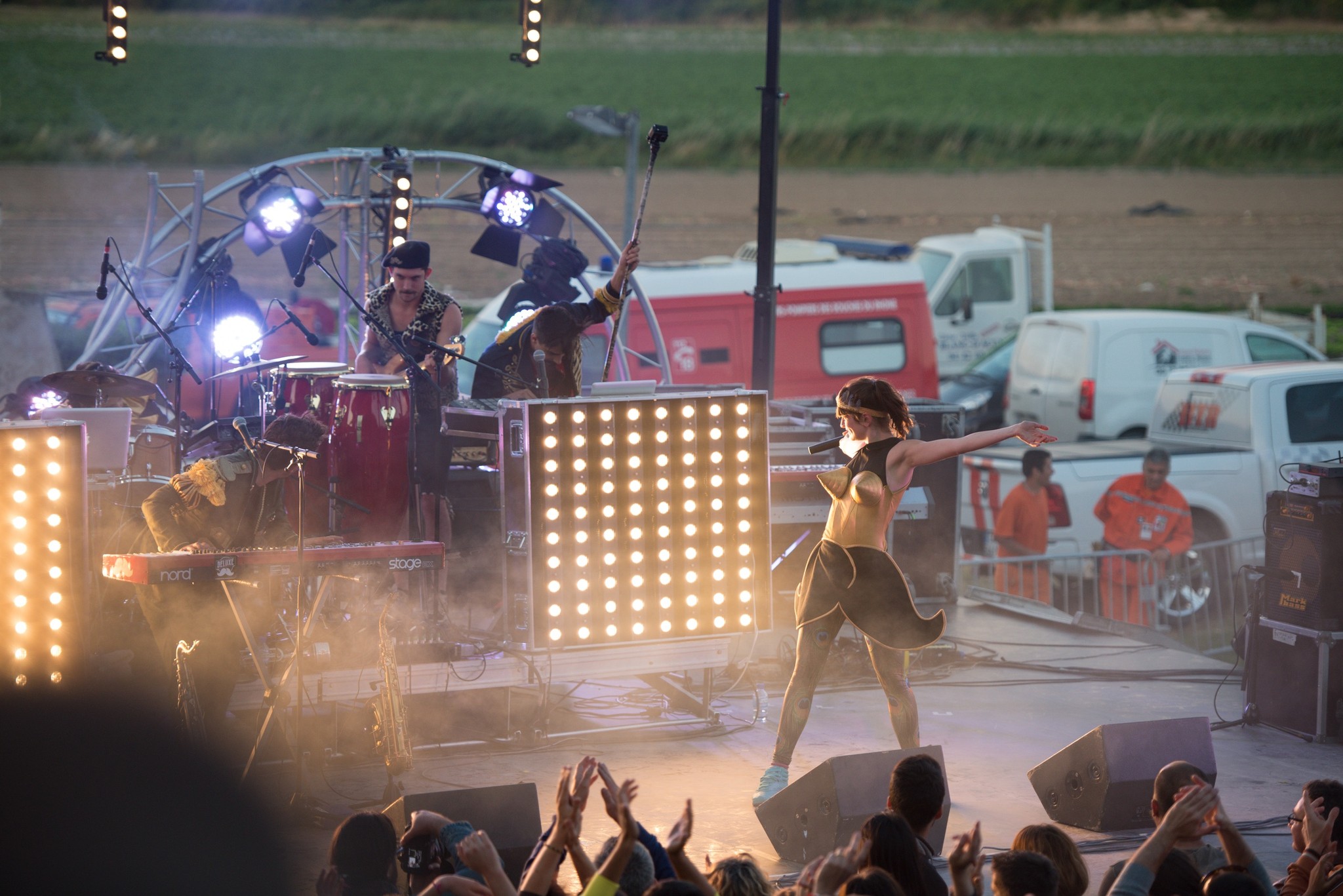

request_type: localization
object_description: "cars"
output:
[939,333,1016,445]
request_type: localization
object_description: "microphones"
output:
[808,436,844,454]
[135,326,180,345]
[279,301,319,346]
[232,417,260,460]
[96,239,111,301]
[293,230,316,287]
[1244,563,1296,581]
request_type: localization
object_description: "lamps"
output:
[234,157,339,282]
[4,376,68,421]
[168,228,268,367]
[0,417,93,690]
[490,384,777,650]
[467,164,566,268]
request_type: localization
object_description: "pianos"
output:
[100,541,448,798]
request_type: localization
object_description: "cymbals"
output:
[38,365,158,400]
[197,352,309,382]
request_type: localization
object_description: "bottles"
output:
[753,682,768,721]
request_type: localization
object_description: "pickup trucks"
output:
[960,360,1343,630]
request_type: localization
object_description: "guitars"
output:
[372,343,466,379]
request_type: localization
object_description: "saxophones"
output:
[172,638,210,760]
[370,591,415,777]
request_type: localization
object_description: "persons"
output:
[312,751,1343,896]
[126,408,329,751]
[352,240,466,635]
[748,372,1059,811]
[992,448,1055,608]
[1094,446,1196,630]
[470,234,641,396]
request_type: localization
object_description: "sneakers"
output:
[392,589,411,619]
[427,589,448,618]
[751,767,789,806]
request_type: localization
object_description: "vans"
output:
[1000,309,1328,444]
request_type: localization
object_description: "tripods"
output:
[1211,580,1314,743]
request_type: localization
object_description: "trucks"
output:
[908,215,1327,394]
[453,233,940,568]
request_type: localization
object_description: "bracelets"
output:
[1158,546,1172,558]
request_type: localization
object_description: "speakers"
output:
[381,782,542,866]
[755,746,952,866]
[1027,716,1217,833]
[1262,491,1342,632]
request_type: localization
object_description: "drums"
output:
[107,422,185,511]
[269,360,355,537]
[327,372,418,546]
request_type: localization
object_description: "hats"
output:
[382,241,432,268]
